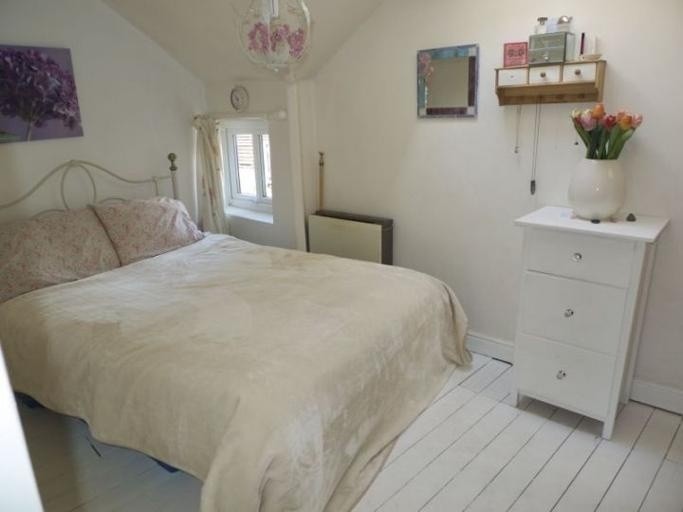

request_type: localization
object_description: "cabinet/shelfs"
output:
[506,206,670,440]
[494,60,606,104]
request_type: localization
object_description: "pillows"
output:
[2,195,205,301]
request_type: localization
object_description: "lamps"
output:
[234,1,312,73]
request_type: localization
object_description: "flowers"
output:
[570,103,643,160]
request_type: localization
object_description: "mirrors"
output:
[416,44,478,119]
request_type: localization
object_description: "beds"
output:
[0,152,452,510]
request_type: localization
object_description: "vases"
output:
[568,160,625,219]
